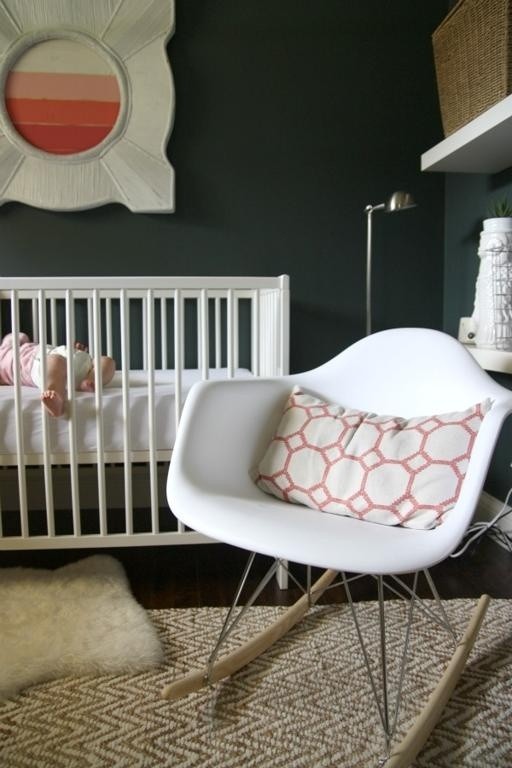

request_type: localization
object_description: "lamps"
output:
[365,191,415,334]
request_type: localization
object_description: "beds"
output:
[0,274,292,590]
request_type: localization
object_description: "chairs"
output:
[166,325,512,768]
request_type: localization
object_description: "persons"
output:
[0,330,119,419]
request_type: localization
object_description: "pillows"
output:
[255,386,486,529]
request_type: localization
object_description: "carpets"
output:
[0,598,512,768]
